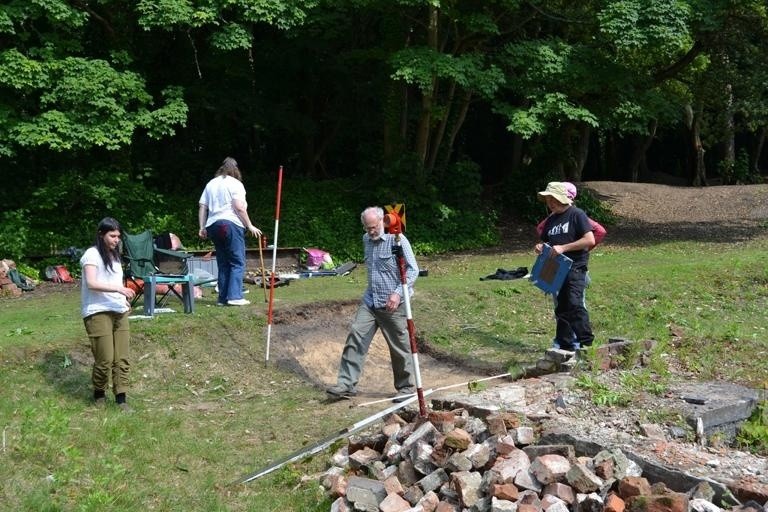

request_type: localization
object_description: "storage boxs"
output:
[186,256,218,278]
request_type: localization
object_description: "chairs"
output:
[121,228,194,316]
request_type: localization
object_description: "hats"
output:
[536,181,578,205]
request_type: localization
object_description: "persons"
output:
[534,182,607,354]
[199,157,263,305]
[80,217,136,411]
[326,206,420,402]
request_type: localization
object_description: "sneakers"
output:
[326,386,358,399]
[392,387,417,403]
[216,298,251,307]
[119,403,134,412]
[93,396,106,408]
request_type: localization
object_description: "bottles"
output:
[262,235,267,248]
